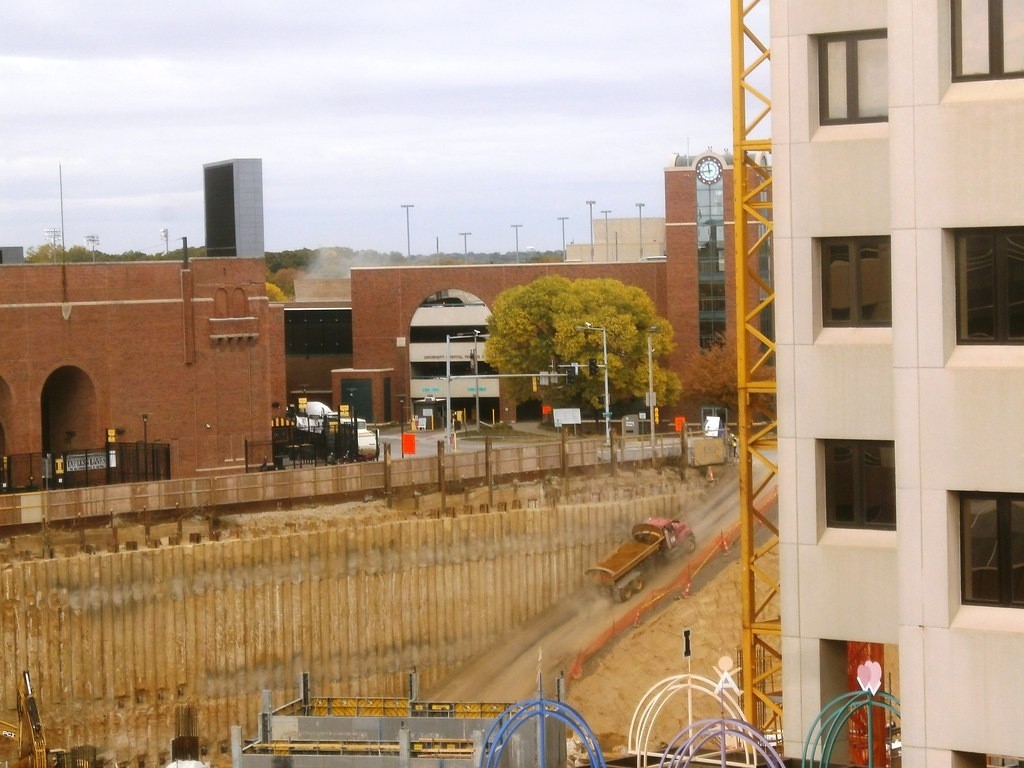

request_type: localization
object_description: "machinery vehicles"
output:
[294,400,380,462]
[0,668,48,768]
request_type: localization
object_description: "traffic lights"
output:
[589,357,596,376]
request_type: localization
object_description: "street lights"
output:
[401,204,414,262]
[446,333,491,454]
[586,200,596,262]
[510,224,523,263]
[574,325,612,448]
[635,203,646,259]
[557,217,569,263]
[459,232,472,265]
[601,210,612,262]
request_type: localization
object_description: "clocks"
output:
[696,156,724,184]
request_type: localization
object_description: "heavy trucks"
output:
[584,516,697,603]
